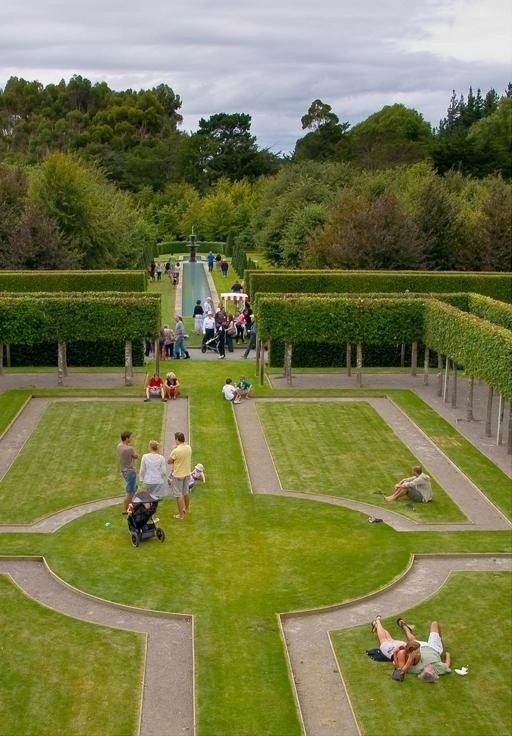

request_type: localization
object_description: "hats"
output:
[207,311,212,316]
[194,463,204,471]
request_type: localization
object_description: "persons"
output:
[221,260,228,277]
[191,462,205,483]
[142,371,167,402]
[383,464,433,505]
[395,617,452,682]
[137,439,169,523]
[221,378,241,402]
[147,252,180,286]
[215,253,221,271]
[144,295,260,358]
[231,280,243,304]
[164,371,180,399]
[115,430,138,514]
[207,249,214,271]
[165,431,192,518]
[236,375,253,399]
[371,614,421,673]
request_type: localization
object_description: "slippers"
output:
[121,504,159,524]
[183,508,191,513]
[172,514,185,520]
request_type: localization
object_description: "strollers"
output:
[202,333,220,352]
[126,493,166,549]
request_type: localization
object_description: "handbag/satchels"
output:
[392,668,405,680]
[170,330,177,341]
[365,648,388,660]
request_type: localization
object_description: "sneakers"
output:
[161,396,177,401]
[234,400,240,403]
[238,395,242,398]
[144,399,151,402]
[245,396,251,399]
[240,354,247,358]
[218,355,224,358]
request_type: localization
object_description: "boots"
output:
[184,351,190,359]
[174,354,180,359]
